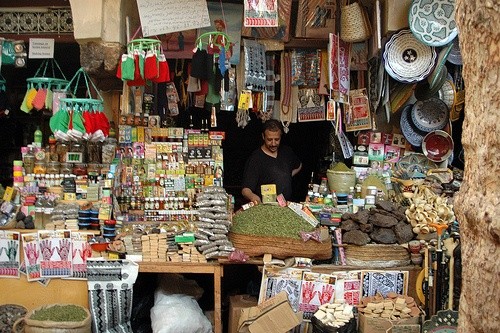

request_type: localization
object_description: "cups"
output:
[13,160,24,187]
[20,146,34,174]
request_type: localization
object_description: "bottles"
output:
[188,115,194,128]
[161,107,167,127]
[117,155,222,222]
[320,177,385,214]
[29,142,105,190]
[200,119,208,134]
[34,126,42,142]
[34,194,56,229]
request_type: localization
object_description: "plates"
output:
[408,0,459,46]
[400,104,428,146]
[422,130,455,162]
[381,27,438,83]
[424,35,463,96]
[410,96,449,132]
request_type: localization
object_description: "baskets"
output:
[340,0,373,43]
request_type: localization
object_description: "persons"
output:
[240,121,302,202]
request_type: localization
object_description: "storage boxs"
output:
[229,295,300,333]
[359,310,421,333]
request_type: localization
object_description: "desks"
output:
[89,260,224,333]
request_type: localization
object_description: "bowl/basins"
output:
[77,211,115,253]
[434,79,457,111]
[390,83,417,114]
